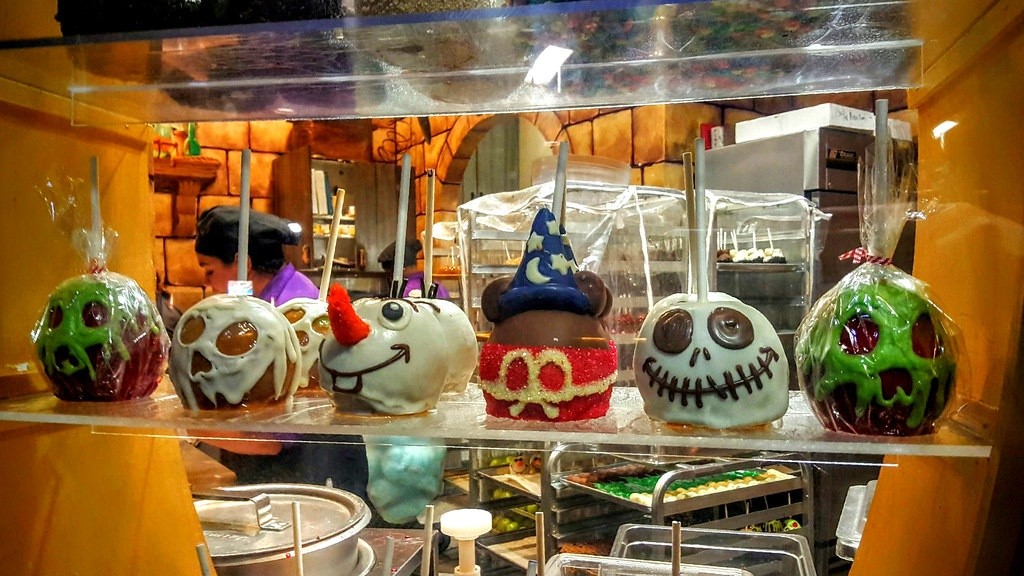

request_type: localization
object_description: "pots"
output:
[192,482,372,576]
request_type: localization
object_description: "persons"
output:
[193,205,369,505]
[376,236,449,304]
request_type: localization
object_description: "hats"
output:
[194,204,301,270]
[378,238,422,267]
